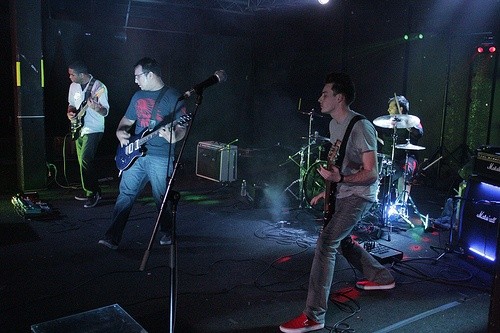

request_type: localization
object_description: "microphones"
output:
[179,69,227,100]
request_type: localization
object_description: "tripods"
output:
[420,31,497,264]
[280,113,428,241]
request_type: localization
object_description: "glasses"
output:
[134,72,146,80]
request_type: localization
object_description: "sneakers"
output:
[356,281,395,289]
[280,313,325,333]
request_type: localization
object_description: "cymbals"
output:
[373,114,420,128]
[298,136,330,141]
[391,143,426,150]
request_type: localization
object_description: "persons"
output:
[279,74,426,333]
[67,62,109,208]
[97,57,188,251]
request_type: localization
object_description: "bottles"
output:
[241,180,246,196]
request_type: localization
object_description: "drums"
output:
[377,154,392,177]
[303,160,327,212]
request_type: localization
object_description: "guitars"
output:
[319,139,342,236]
[115,112,191,171]
[71,88,104,141]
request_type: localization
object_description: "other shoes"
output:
[84,191,99,207]
[160,233,171,245]
[75,191,101,200]
[98,239,118,250]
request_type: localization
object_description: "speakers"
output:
[195,141,238,182]
